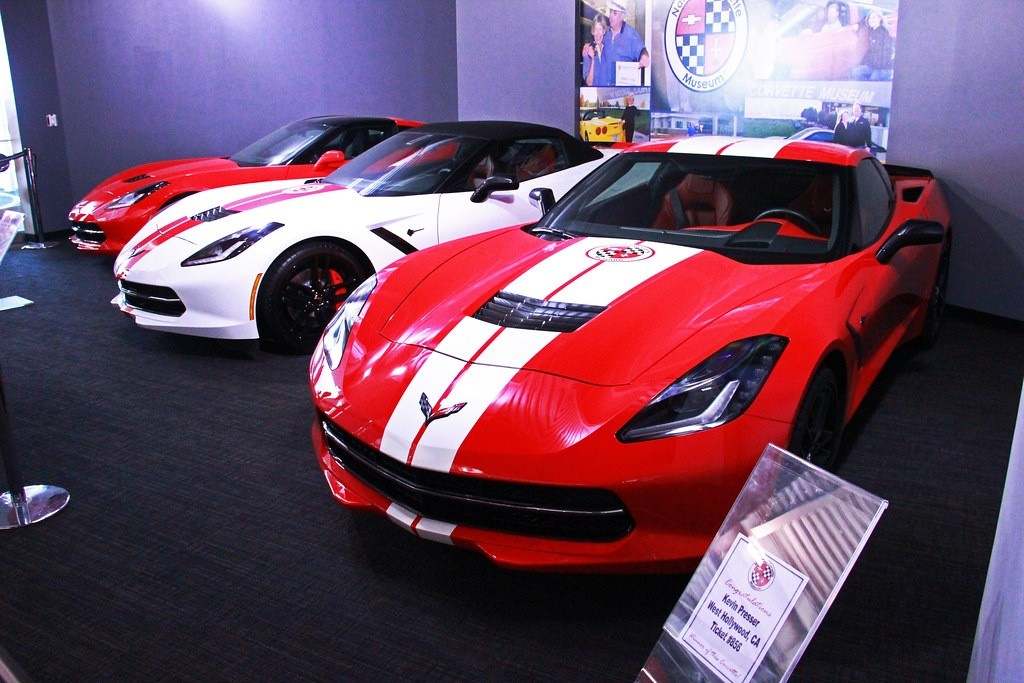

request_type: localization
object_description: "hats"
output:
[606,0,628,12]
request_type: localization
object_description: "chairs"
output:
[345,128,371,161]
[467,151,495,188]
[790,170,839,241]
[651,172,735,230]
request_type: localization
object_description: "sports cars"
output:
[579,109,628,143]
[66,116,411,255]
[306,137,953,570]
[762,126,884,157]
[112,120,603,352]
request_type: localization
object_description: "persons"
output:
[618,93,641,143]
[801,4,842,37]
[687,122,705,137]
[854,9,892,80]
[582,0,651,87]
[832,108,852,146]
[851,101,873,152]
